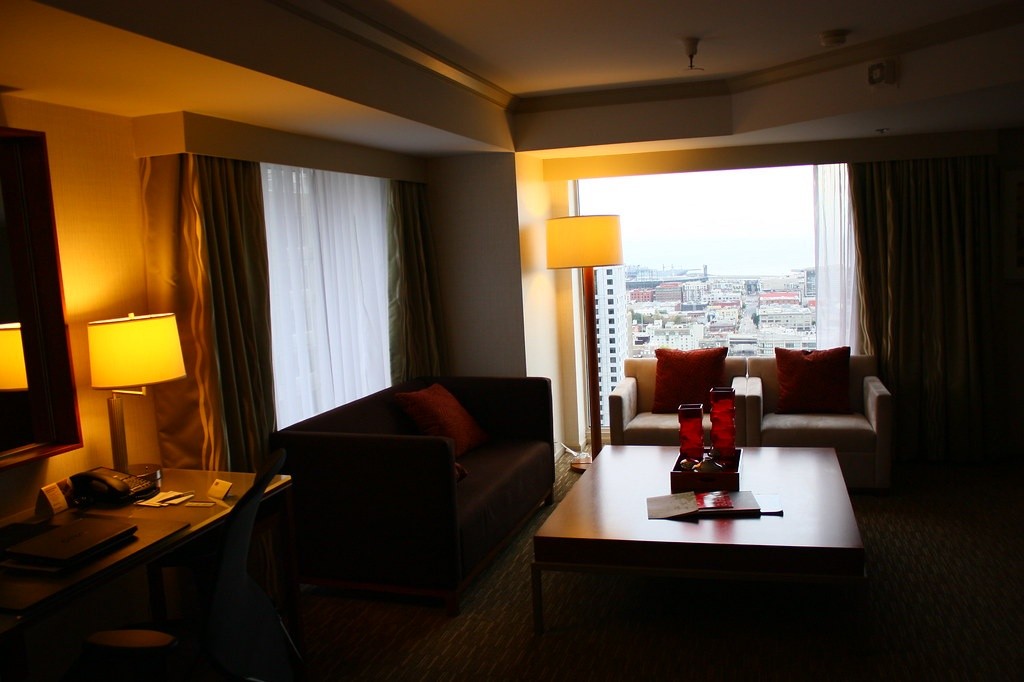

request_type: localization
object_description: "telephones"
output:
[69,466,160,507]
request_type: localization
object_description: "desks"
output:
[0,467,294,682]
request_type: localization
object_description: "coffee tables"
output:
[532,445,865,654]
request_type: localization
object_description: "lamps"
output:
[0,322,30,392]
[546,215,623,473]
[87,312,188,483]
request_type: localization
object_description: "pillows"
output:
[774,346,851,414]
[394,384,490,459]
[651,347,728,414]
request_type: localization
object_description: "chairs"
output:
[127,448,305,682]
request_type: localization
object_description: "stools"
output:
[81,628,179,682]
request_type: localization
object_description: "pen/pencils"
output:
[157,490,193,503]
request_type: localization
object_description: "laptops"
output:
[7,517,137,570]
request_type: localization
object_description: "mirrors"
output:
[0,125,84,472]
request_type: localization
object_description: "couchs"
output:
[269,376,556,605]
[746,356,893,494]
[609,357,746,446]
